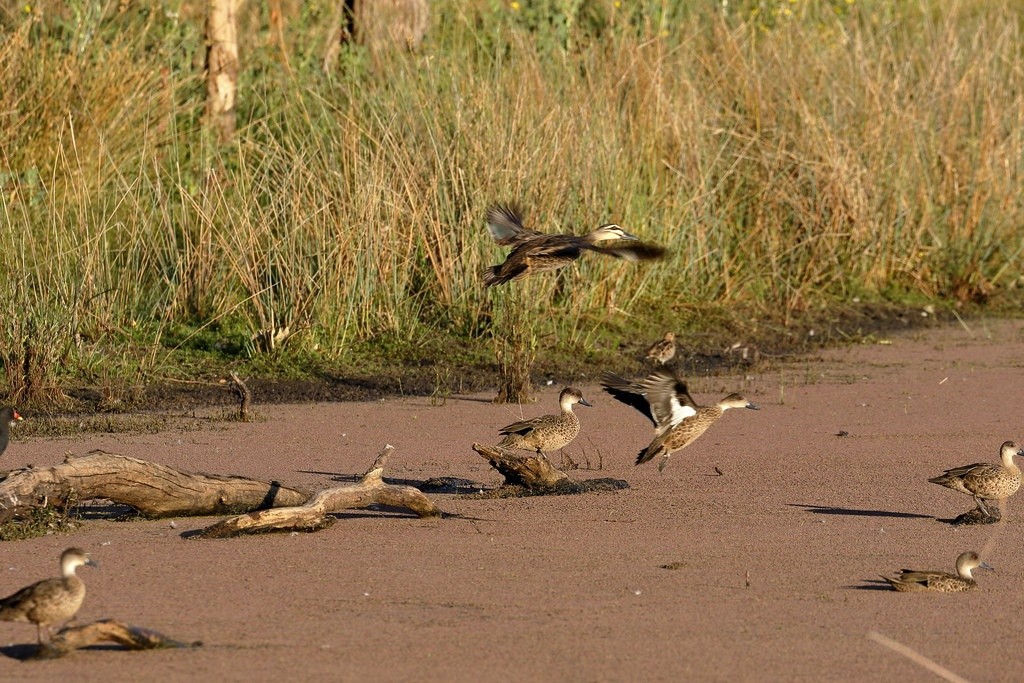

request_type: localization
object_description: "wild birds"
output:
[493,387,592,460]
[479,199,666,289]
[877,551,994,592]
[598,365,761,472]
[927,440,1024,518]
[0,547,97,649]
[642,330,681,367]
